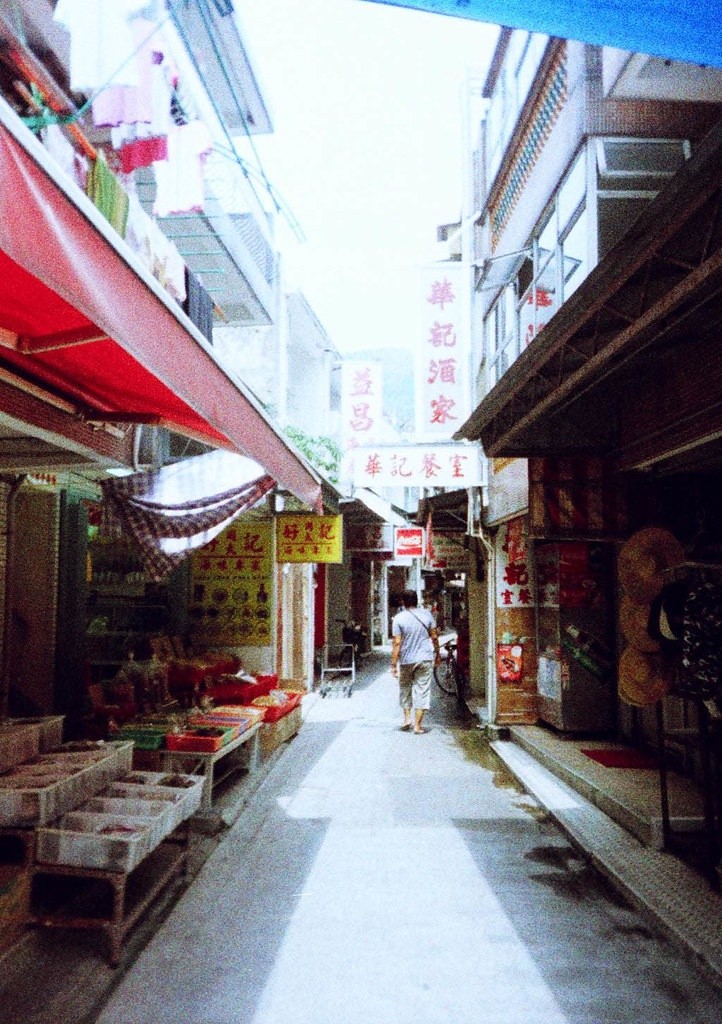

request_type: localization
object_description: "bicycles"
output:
[335,618,367,675]
[433,638,467,707]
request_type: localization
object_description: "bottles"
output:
[563,622,616,679]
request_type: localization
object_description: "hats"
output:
[617,527,688,707]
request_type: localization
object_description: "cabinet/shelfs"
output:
[529,534,620,736]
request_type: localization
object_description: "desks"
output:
[163,722,265,815]
[132,746,168,773]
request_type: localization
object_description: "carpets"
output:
[585,747,672,772]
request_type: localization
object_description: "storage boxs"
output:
[0,655,302,873]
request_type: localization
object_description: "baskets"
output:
[343,627,360,645]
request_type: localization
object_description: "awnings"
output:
[0,90,324,514]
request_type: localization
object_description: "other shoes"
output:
[414,728,426,734]
[398,724,411,731]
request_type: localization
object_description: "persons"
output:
[390,591,440,733]
[397,599,406,613]
[422,598,439,616]
